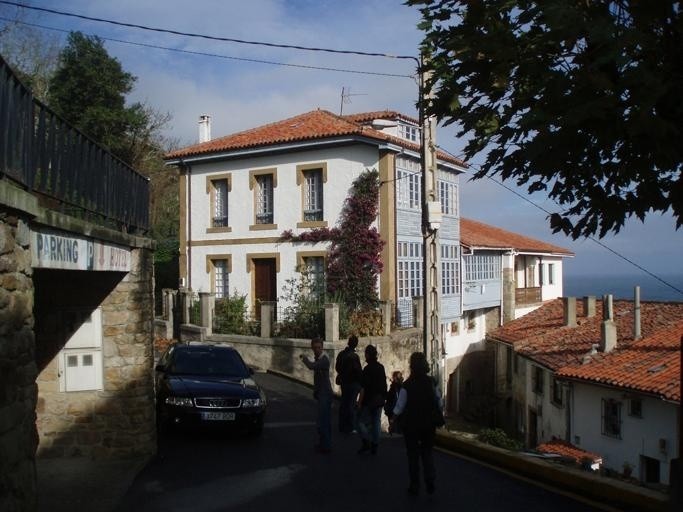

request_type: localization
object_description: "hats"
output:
[155,340,267,432]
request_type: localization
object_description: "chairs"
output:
[425,483,435,495]
[357,447,370,454]
[371,442,378,455]
[407,487,419,496]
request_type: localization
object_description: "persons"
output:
[299,338,333,453]
[385,371,403,429]
[334,335,361,433]
[390,352,445,495]
[354,345,385,454]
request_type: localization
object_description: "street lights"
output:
[335,351,343,385]
[430,376,444,427]
[376,431,403,464]
[367,395,386,411]
